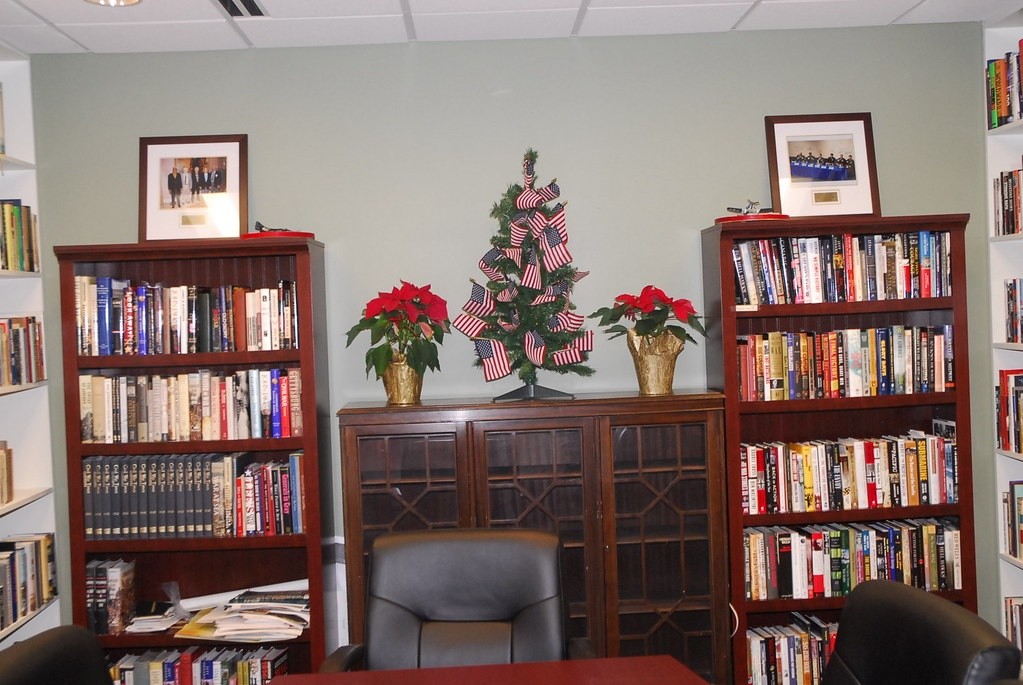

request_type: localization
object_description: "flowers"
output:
[586,284,712,345]
[345,278,452,381]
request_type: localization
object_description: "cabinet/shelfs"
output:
[52,237,338,673]
[0,153,59,640]
[986,118,1023,571]
[701,213,978,685]
[335,388,734,685]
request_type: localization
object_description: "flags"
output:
[452,159,594,383]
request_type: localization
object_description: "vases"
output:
[381,355,423,405]
[624,331,683,395]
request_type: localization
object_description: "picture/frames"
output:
[138,133,249,242]
[764,112,882,218]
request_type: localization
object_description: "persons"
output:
[795,151,854,170]
[167,165,221,208]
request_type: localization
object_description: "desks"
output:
[266,654,708,685]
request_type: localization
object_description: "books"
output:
[79,367,304,444]
[743,518,963,602]
[73,275,301,357]
[1001,480,1023,564]
[0,81,6,155]
[0,532,59,631]
[985,39,1023,130]
[735,324,955,403]
[1004,596,1023,651]
[732,230,953,311]
[0,315,48,386]
[104,645,288,685]
[993,153,1023,239]
[994,368,1023,455]
[745,610,838,685]
[1003,276,1023,345]
[0,439,14,507]
[0,198,40,274]
[86,558,312,643]
[739,417,959,515]
[81,449,306,542]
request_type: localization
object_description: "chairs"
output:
[820,579,1021,685]
[0,625,114,685]
[319,527,598,675]
